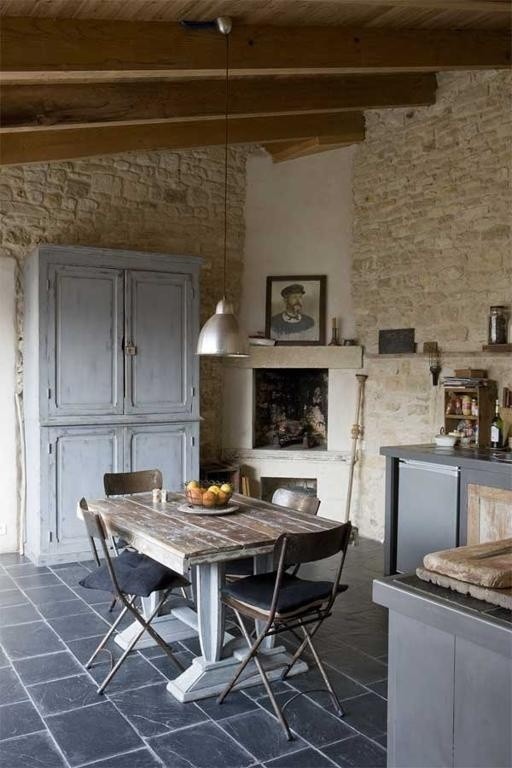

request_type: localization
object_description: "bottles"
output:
[151,487,168,503]
[490,398,503,448]
[488,305,508,345]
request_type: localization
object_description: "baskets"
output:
[184,479,233,509]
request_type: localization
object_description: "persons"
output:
[271,284,314,334]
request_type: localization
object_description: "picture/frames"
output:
[264,275,327,346]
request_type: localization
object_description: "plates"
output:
[178,502,240,516]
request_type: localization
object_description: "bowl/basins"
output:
[434,433,457,447]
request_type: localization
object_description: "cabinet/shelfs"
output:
[442,387,496,449]
[23,243,201,566]
[380,443,512,577]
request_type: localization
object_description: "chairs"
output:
[103,467,189,612]
[78,496,185,695]
[223,487,320,643]
[215,520,352,742]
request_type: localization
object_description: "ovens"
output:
[393,458,462,580]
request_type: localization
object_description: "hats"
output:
[281,284,305,295]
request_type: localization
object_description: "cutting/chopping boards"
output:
[414,534,512,610]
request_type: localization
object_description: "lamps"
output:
[195,16,251,358]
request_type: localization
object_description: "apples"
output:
[186,480,232,506]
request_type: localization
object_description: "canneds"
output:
[461,395,476,416]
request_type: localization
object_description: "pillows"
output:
[79,549,191,597]
[272,486,319,515]
[219,570,349,618]
